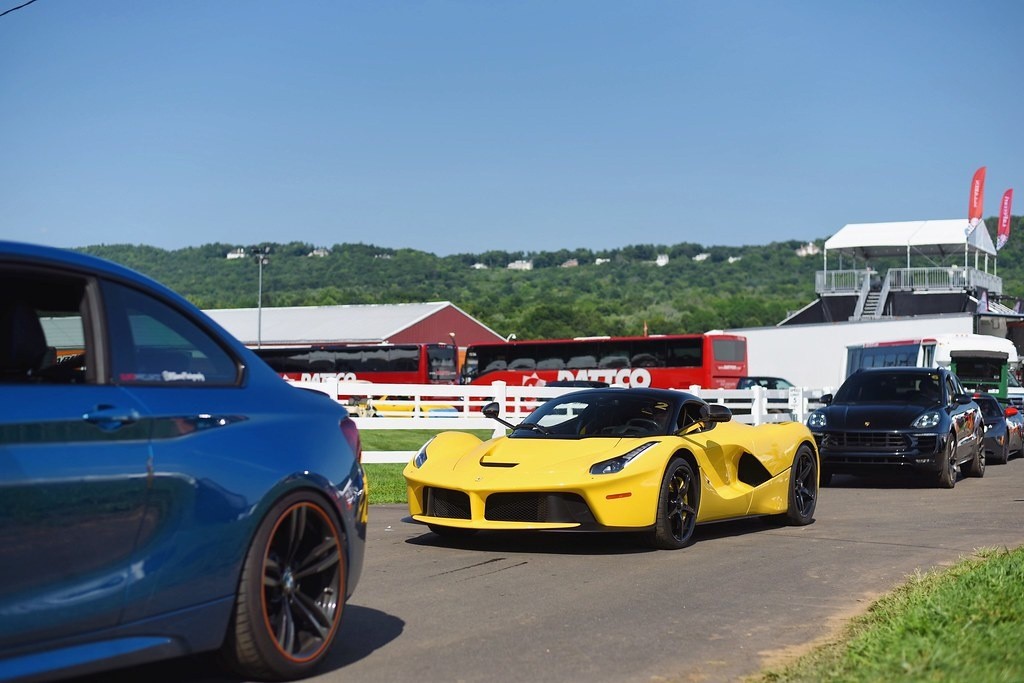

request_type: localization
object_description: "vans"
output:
[1007,370,1024,414]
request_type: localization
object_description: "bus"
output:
[843,334,1020,406]
[453,331,751,412]
[243,340,461,417]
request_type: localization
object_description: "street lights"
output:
[251,245,271,348]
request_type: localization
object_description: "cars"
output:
[730,375,796,413]
[0,238,371,683]
[949,351,1024,463]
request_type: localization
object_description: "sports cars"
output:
[399,383,822,549]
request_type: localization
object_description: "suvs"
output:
[806,364,986,489]
